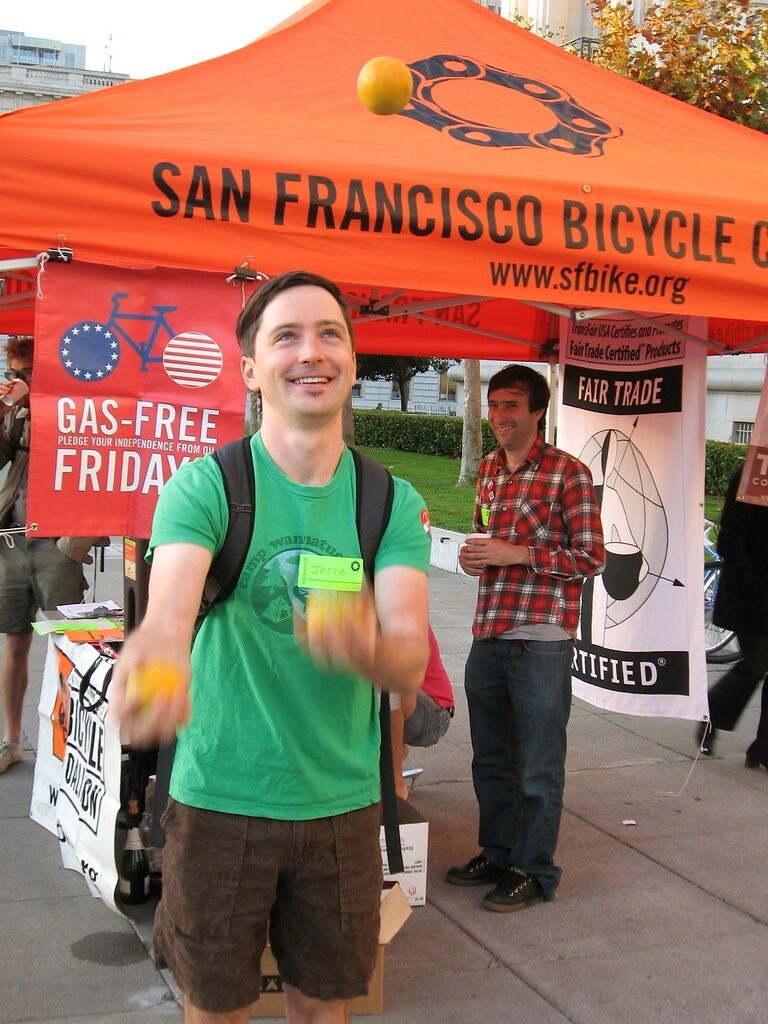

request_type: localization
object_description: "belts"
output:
[445,704,455,718]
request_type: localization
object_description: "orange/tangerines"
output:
[357,57,413,115]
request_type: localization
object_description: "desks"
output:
[34,609,178,901]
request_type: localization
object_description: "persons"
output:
[445,365,607,913]
[106,272,432,1023]
[388,618,456,800]
[0,337,103,775]
[696,461,768,772]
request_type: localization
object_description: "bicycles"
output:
[703,516,743,663]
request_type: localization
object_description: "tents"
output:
[1,0,767,448]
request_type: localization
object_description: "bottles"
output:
[119,800,150,905]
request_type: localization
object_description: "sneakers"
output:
[483,866,545,911]
[0,740,21,774]
[446,850,509,886]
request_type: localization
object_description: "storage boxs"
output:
[248,880,413,1018]
[378,794,428,908]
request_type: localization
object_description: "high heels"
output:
[697,723,714,756]
[744,754,768,769]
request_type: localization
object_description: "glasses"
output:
[3,369,32,382]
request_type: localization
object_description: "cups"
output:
[466,533,491,567]
[2,379,29,406]
